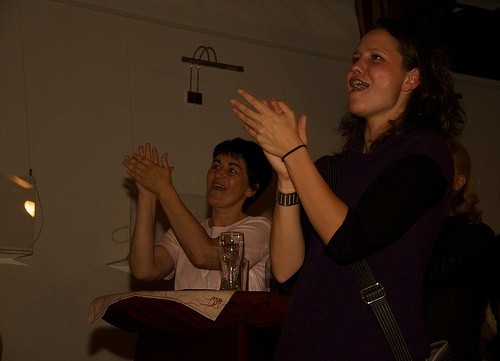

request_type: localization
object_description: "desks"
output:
[86,289,291,361]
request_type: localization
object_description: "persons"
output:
[231,16,467,361]
[424,140,500,361]
[122,138,271,291]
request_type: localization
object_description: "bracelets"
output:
[274,189,300,206]
[281,145,307,161]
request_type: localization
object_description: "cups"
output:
[219,232,244,289]
